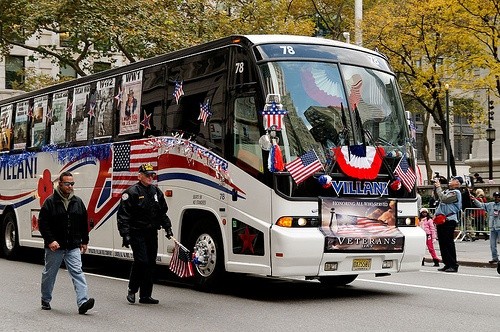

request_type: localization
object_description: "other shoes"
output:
[485,235,489,240]
[78,298,95,314]
[489,260,499,263]
[438,266,458,272]
[41,301,51,310]
[473,236,479,241]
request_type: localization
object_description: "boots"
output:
[433,258,439,266]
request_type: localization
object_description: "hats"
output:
[419,208,428,213]
[451,176,463,184]
[127,87,134,95]
[139,164,156,175]
[493,192,500,196]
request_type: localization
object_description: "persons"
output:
[38,172,95,314]
[418,208,439,267]
[461,185,489,241]
[124,88,137,118]
[469,191,500,264]
[433,176,464,273]
[96,97,113,136]
[117,164,173,304]
[474,173,484,183]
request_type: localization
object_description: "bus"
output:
[0,34,431,294]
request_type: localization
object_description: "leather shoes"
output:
[127,290,136,303]
[139,297,159,304]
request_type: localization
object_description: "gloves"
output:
[431,239,436,243]
[165,230,174,240]
[122,237,130,248]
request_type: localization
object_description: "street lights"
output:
[485,123,496,180]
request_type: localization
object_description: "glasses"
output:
[495,197,500,198]
[144,174,154,178]
[62,181,75,186]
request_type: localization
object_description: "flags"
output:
[394,157,417,193]
[169,244,194,278]
[285,150,323,185]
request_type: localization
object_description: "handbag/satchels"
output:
[433,213,446,225]
